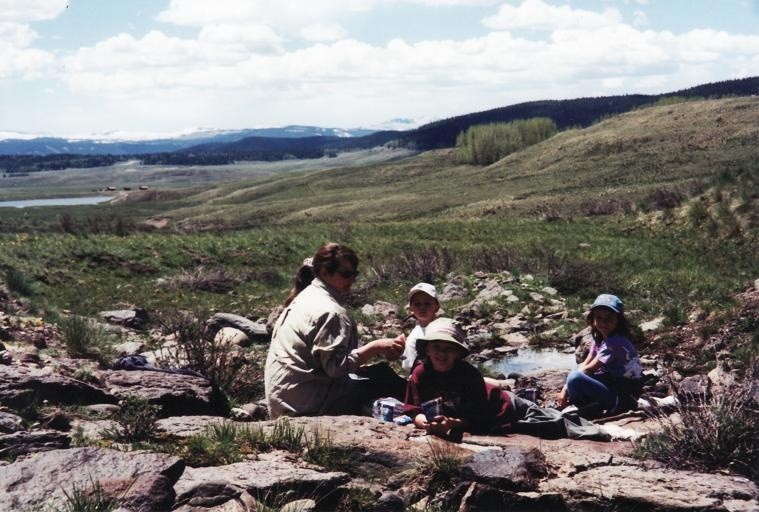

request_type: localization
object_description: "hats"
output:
[408,283,439,306]
[416,318,472,359]
[588,293,624,316]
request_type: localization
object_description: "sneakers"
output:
[560,400,604,421]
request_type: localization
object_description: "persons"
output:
[265,243,406,420]
[266,257,315,334]
[403,317,538,434]
[400,282,440,374]
[557,294,643,421]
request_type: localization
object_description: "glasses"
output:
[329,266,360,280]
